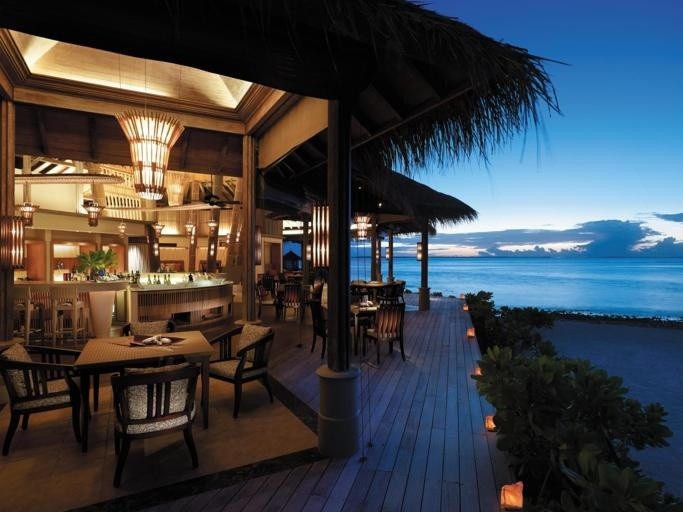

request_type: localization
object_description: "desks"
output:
[76,330,216,456]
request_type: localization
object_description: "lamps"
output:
[114,59,183,201]
[81,179,105,227]
[13,183,39,227]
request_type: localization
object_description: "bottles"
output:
[188,272,194,282]
[130,269,172,286]
[62,272,71,281]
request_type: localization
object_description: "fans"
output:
[184,173,240,208]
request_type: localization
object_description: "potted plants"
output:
[77,248,116,282]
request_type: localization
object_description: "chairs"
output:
[207,322,275,420]
[12,286,85,347]
[0,342,99,456]
[255,273,406,365]
[110,361,202,486]
[120,319,177,336]
